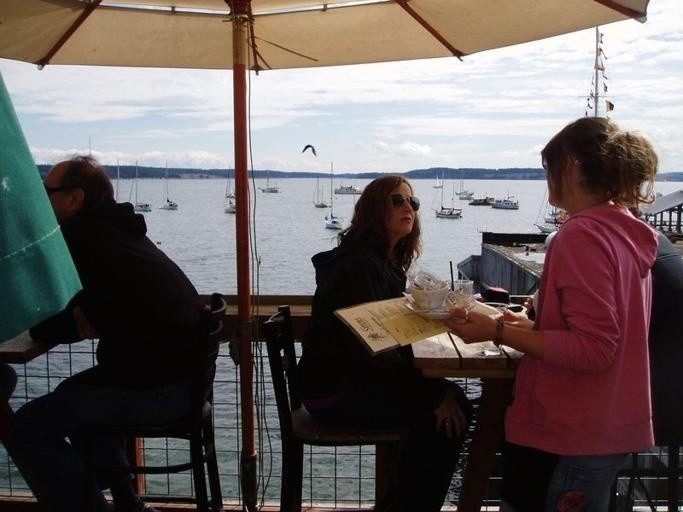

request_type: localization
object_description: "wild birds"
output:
[302,145,316,156]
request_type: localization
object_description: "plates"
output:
[417,312,453,321]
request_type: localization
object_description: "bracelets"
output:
[492,318,504,344]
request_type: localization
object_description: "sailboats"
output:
[432,170,520,218]
[533,180,569,233]
[224,164,280,214]
[157,160,177,210]
[127,160,153,211]
[311,161,362,230]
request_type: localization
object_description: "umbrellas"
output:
[0,1,654,511]
[0,70,84,339]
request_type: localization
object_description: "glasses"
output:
[387,192,420,211]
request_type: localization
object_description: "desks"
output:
[403,280,617,512]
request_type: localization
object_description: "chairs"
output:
[109,291,228,512]
[615,428,683,512]
[263,304,425,512]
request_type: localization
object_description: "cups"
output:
[447,280,476,307]
[411,280,448,309]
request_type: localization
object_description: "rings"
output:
[464,309,468,320]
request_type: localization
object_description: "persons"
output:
[289,169,469,511]
[14,155,215,509]
[496,208,683,512]
[439,114,659,511]
